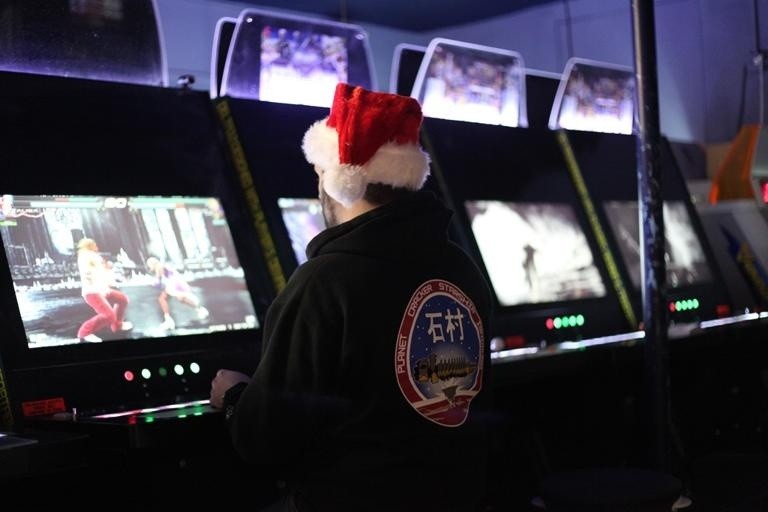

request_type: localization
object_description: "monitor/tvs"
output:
[0,192,262,353]
[460,192,613,314]
[274,192,330,269]
[597,191,719,295]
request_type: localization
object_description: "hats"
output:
[300,83,434,209]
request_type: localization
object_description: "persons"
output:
[210,82,500,511]
[72,237,135,343]
[142,254,211,330]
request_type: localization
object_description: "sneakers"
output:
[78,333,103,344]
[197,308,208,319]
[113,322,131,332]
[161,319,174,330]
